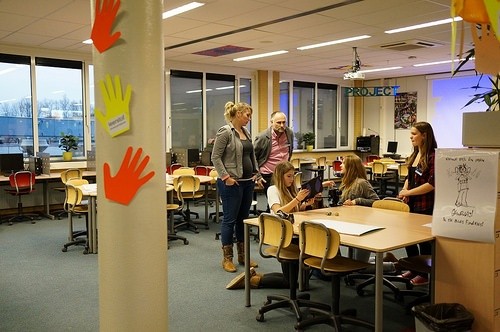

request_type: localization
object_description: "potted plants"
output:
[303,132,315,152]
[58,132,79,161]
[295,131,303,149]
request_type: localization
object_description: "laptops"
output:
[0,154,23,176]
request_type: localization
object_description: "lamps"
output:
[344,47,365,80]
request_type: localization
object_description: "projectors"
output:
[343,72,366,80]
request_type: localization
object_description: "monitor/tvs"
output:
[387,141,398,154]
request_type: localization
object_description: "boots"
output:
[238,242,258,268]
[222,245,237,272]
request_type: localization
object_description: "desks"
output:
[80,183,97,254]
[243,205,436,332]
[193,175,220,226]
[0,171,96,220]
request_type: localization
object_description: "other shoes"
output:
[410,275,427,284]
[227,266,256,289]
[386,252,398,262]
[402,271,412,277]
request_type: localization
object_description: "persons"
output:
[394,121,439,285]
[211,100,260,272]
[225,159,310,290]
[335,151,406,275]
[252,110,294,216]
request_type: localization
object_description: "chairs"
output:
[4,171,42,226]
[167,156,430,332]
[50,168,89,255]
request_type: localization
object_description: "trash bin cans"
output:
[412,303,474,332]
